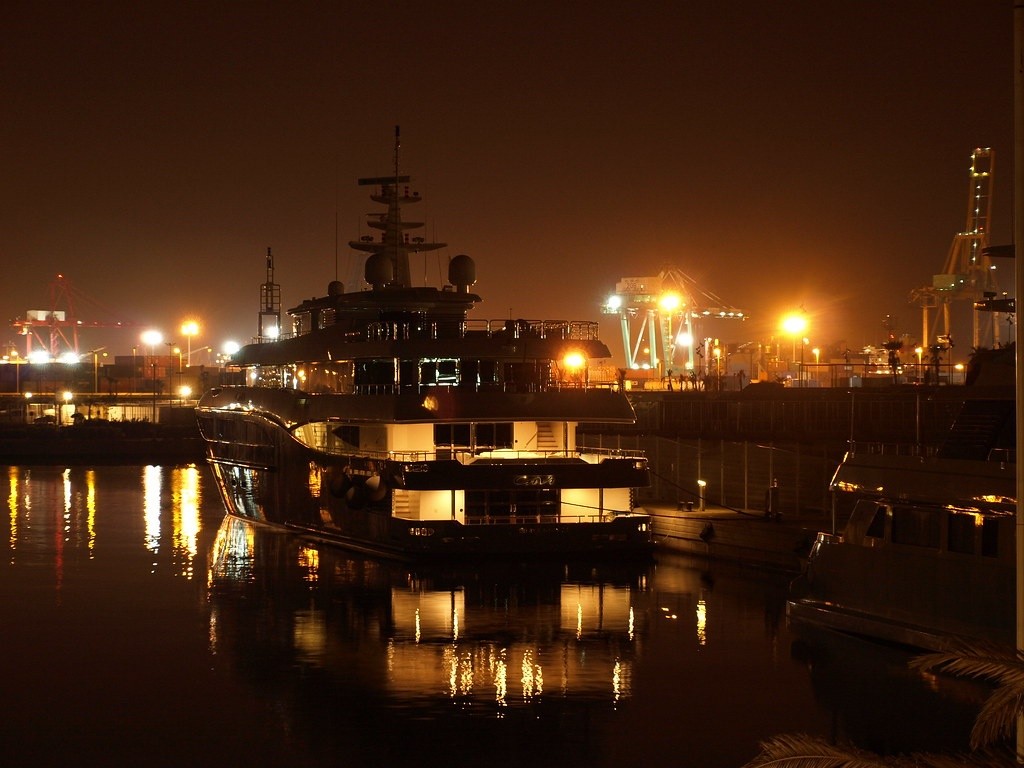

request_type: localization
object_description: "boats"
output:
[779,368,1017,709]
[196,119,660,565]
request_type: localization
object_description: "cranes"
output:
[927,145,1000,351]
[601,253,748,381]
[45,269,141,364]
